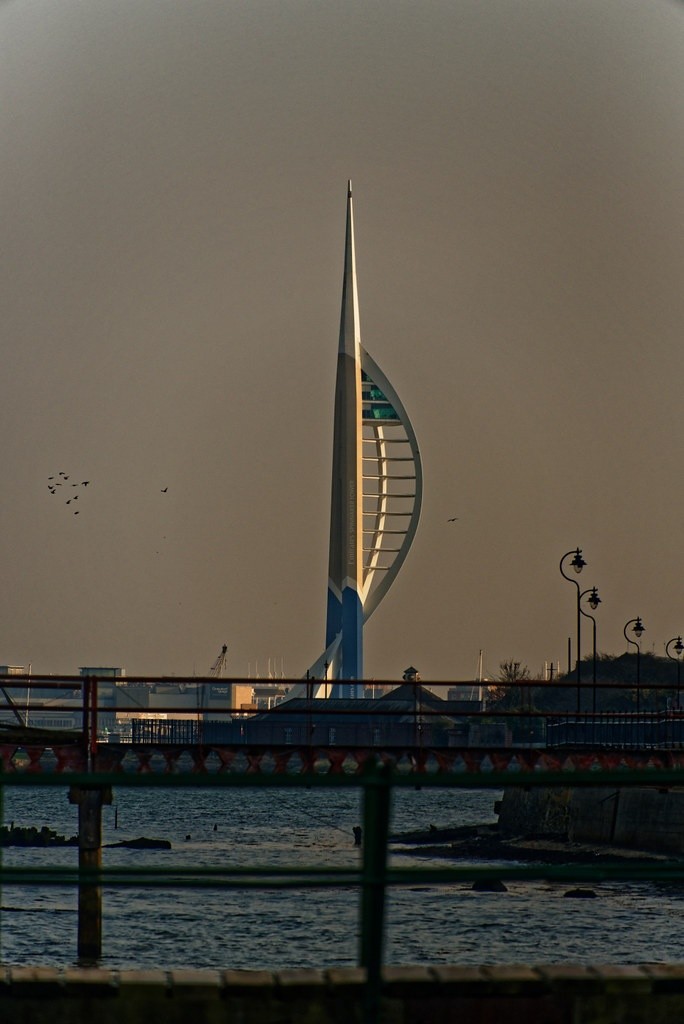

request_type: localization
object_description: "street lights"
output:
[323,660,328,700]
[560,546,588,668]
[623,616,646,654]
[577,585,601,663]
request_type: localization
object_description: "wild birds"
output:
[447,518,459,522]
[47,471,90,515]
[160,487,169,493]
